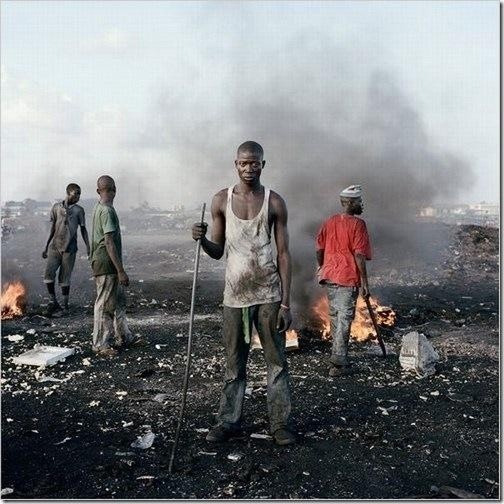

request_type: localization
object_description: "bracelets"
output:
[279,302,292,311]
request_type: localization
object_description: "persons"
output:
[41,181,89,317]
[315,184,372,376]
[190,139,296,446]
[87,173,151,357]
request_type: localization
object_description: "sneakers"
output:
[329,361,360,376]
[274,429,295,446]
[206,424,242,443]
[93,336,149,356]
[42,301,72,317]
